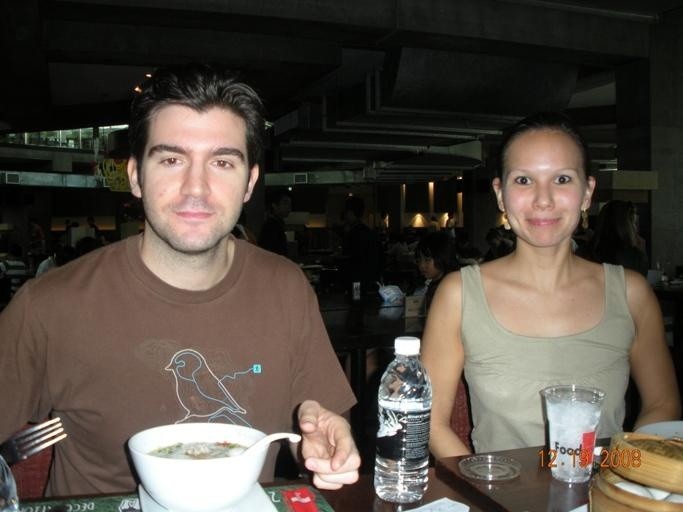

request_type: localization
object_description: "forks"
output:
[1,416,67,466]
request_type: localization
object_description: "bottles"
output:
[373,334,434,502]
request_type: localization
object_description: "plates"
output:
[631,420,682,441]
[137,480,279,512]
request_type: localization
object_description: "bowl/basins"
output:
[124,422,268,512]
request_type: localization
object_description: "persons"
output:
[0,188,655,325]
[0,57,363,493]
[413,109,681,464]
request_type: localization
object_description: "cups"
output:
[542,383,603,484]
